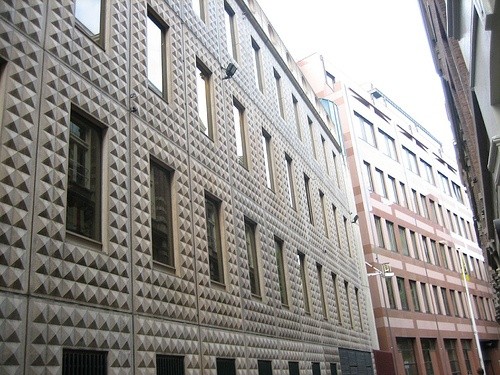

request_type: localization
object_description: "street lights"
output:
[365,261,399,375]
[456,246,488,375]
